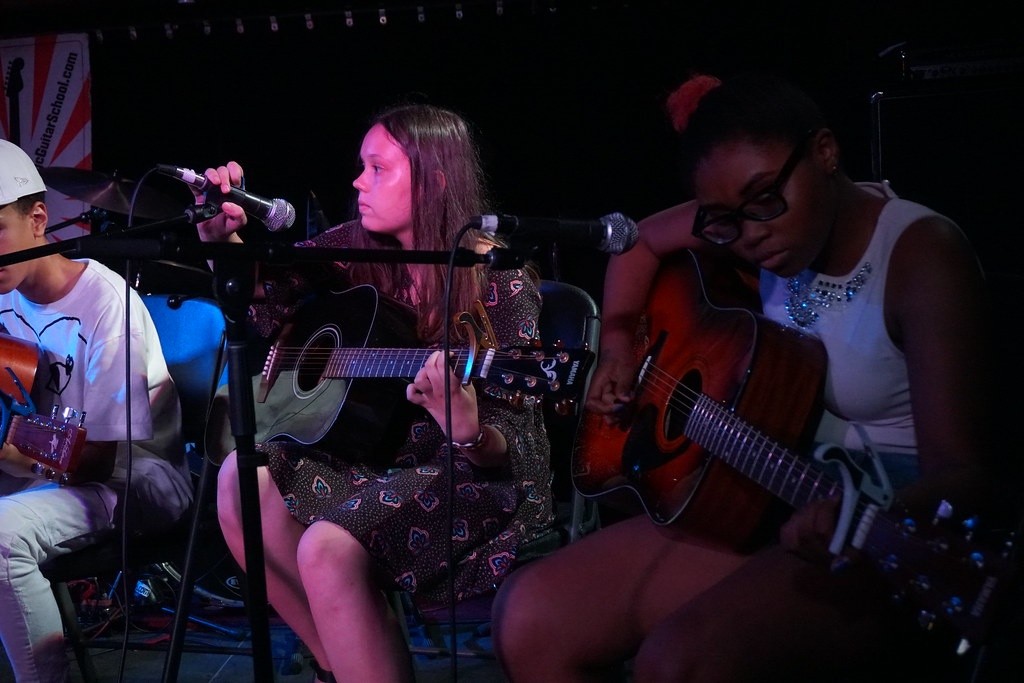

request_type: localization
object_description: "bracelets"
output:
[452,424,486,449]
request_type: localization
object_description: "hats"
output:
[0,139,47,205]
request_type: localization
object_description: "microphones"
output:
[156,165,297,234]
[471,210,640,256]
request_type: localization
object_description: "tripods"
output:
[83,565,245,638]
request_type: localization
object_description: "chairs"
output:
[376,281,602,683]
[44,292,240,683]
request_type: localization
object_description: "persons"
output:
[0,139,193,683]
[490,85,981,682]
[186,105,555,683]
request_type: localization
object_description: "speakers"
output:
[863,87,1007,244]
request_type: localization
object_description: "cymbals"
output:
[42,167,187,220]
[149,257,211,283]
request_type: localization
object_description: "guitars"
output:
[1,328,91,489]
[202,275,598,473]
[568,244,1021,664]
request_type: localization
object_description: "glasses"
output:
[690,128,816,245]
